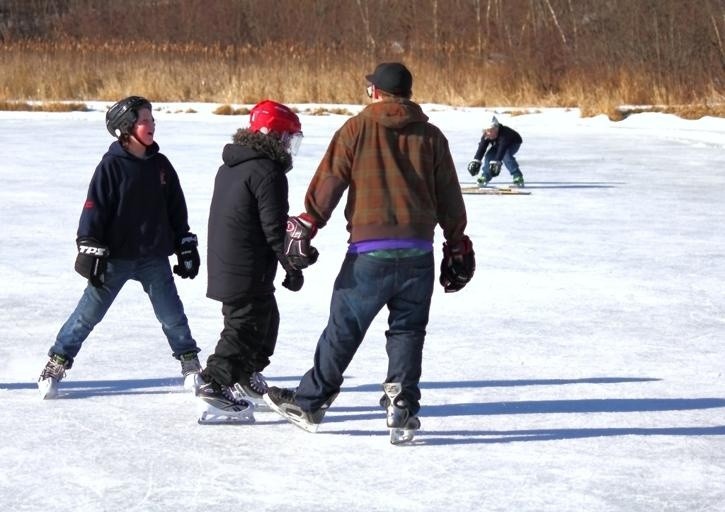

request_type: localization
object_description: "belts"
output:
[366,248,426,258]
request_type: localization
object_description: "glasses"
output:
[367,86,372,97]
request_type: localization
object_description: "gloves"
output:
[174,234,200,279]
[283,213,319,270]
[440,236,475,292]
[468,159,481,175]
[489,160,501,176]
[74,240,109,288]
[282,271,304,291]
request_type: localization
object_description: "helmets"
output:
[106,96,151,137]
[482,115,500,129]
[249,100,303,157]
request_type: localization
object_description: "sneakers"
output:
[37,359,67,384]
[193,371,248,413]
[477,176,487,182]
[513,176,523,183]
[180,352,203,379]
[245,371,269,394]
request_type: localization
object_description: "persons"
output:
[266,61,475,430]
[467,115,523,183]
[194,97,319,413]
[37,95,203,394]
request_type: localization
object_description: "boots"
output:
[382,382,421,429]
[268,386,340,423]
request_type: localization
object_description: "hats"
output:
[366,62,412,96]
